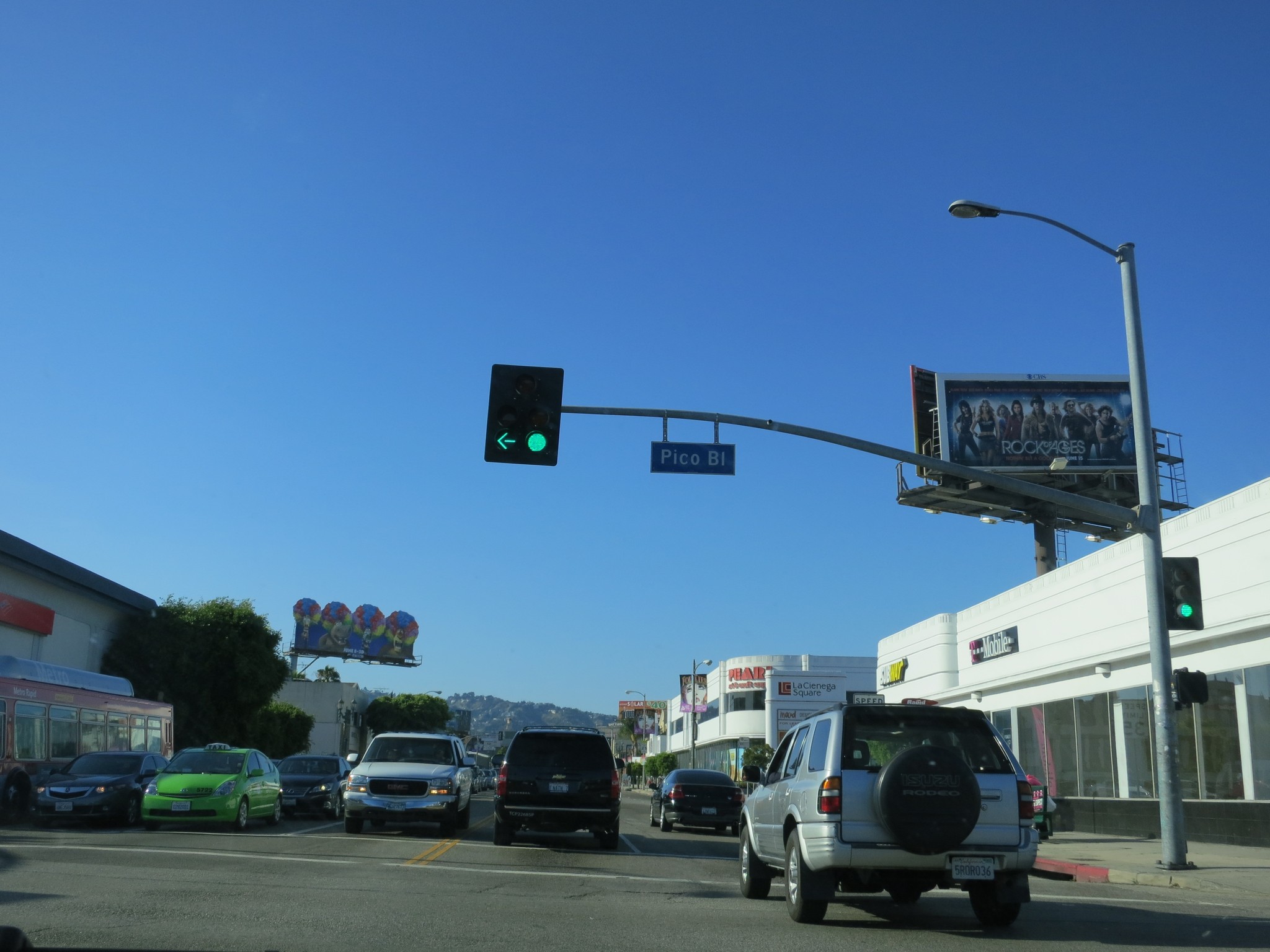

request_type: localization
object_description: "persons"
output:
[952,394,1127,466]
[743,772,747,780]
[435,744,446,760]
[620,706,626,719]
[378,738,405,760]
[768,772,781,784]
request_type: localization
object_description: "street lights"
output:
[945,192,1193,867]
[336,696,359,755]
[596,724,614,750]
[690,659,714,769]
[625,691,646,790]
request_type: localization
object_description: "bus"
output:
[0,653,174,823]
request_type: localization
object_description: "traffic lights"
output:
[482,364,566,467]
[1170,668,1207,706]
[1164,556,1204,631]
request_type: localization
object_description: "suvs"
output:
[344,730,476,838]
[491,725,627,852]
[738,700,1044,934]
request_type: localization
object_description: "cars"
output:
[140,742,283,830]
[470,766,501,793]
[34,749,172,825]
[649,768,747,836]
[275,755,354,822]
